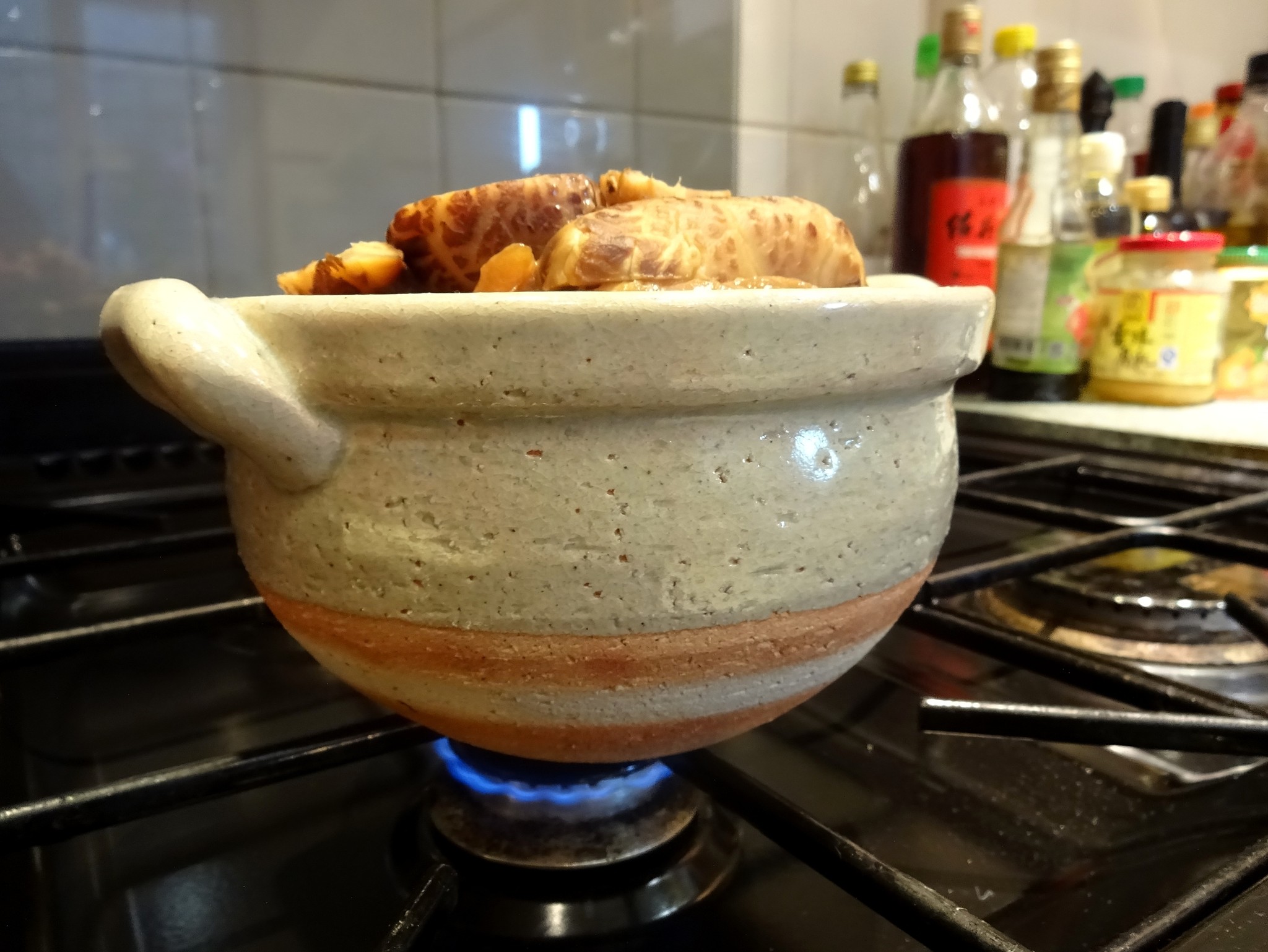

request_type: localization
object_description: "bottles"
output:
[836,2,1266,403]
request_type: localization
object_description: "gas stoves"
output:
[6,331,1267,951]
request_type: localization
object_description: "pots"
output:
[96,276,996,761]
